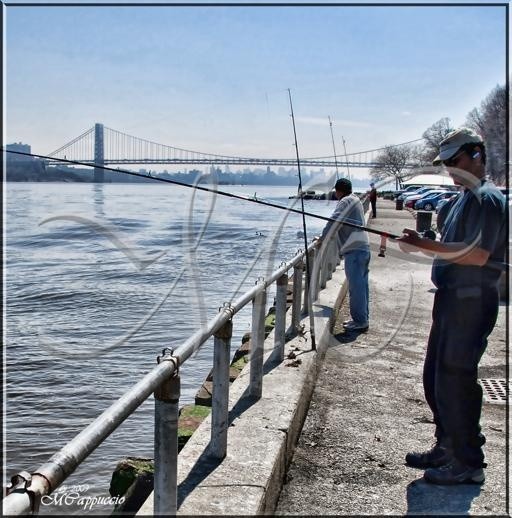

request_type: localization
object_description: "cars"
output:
[382,184,511,214]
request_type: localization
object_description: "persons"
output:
[311,178,371,331]
[369,182,377,218]
[394,128,507,483]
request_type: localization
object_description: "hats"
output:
[332,178,352,188]
[433,128,483,166]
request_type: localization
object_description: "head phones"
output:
[468,146,480,159]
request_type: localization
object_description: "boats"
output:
[306,190,326,199]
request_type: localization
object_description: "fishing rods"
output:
[342,136,350,180]
[289,88,316,351]
[328,114,339,179]
[5,149,435,257]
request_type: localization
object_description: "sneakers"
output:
[343,320,368,325]
[405,445,455,469]
[343,322,369,331]
[424,459,485,485]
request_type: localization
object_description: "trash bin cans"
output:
[416,212,432,232]
[396,200,403,210]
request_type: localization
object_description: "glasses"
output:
[441,148,474,166]
